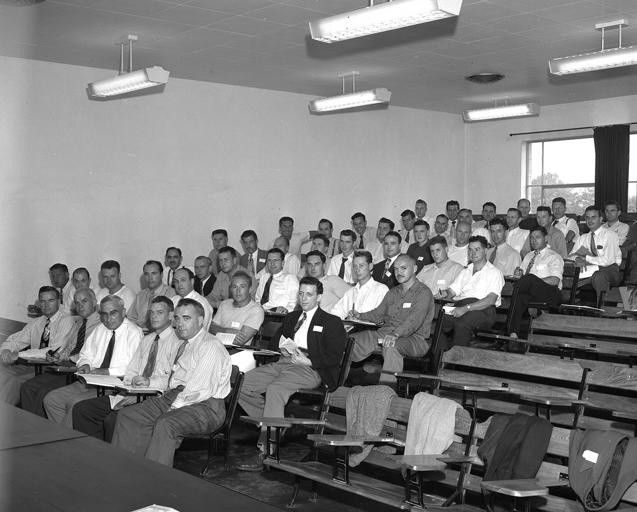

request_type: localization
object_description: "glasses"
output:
[266,258,281,264]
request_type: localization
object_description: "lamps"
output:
[549,18,637,77]
[87,34,171,98]
[463,96,540,122]
[308,0,463,44]
[309,70,392,114]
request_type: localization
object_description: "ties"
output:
[359,235,365,249]
[381,258,391,281]
[167,339,189,390]
[171,272,175,288]
[405,231,409,244]
[142,334,160,379]
[199,281,202,296]
[293,312,307,333]
[260,274,273,305]
[59,287,64,305]
[590,232,598,257]
[69,318,88,357]
[525,251,540,275]
[553,219,559,226]
[39,317,51,349]
[100,330,116,369]
[489,246,498,264]
[338,257,347,280]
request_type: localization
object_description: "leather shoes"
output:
[234,450,277,472]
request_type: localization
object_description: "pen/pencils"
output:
[51,346,61,356]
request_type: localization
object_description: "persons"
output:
[0,197,629,467]
